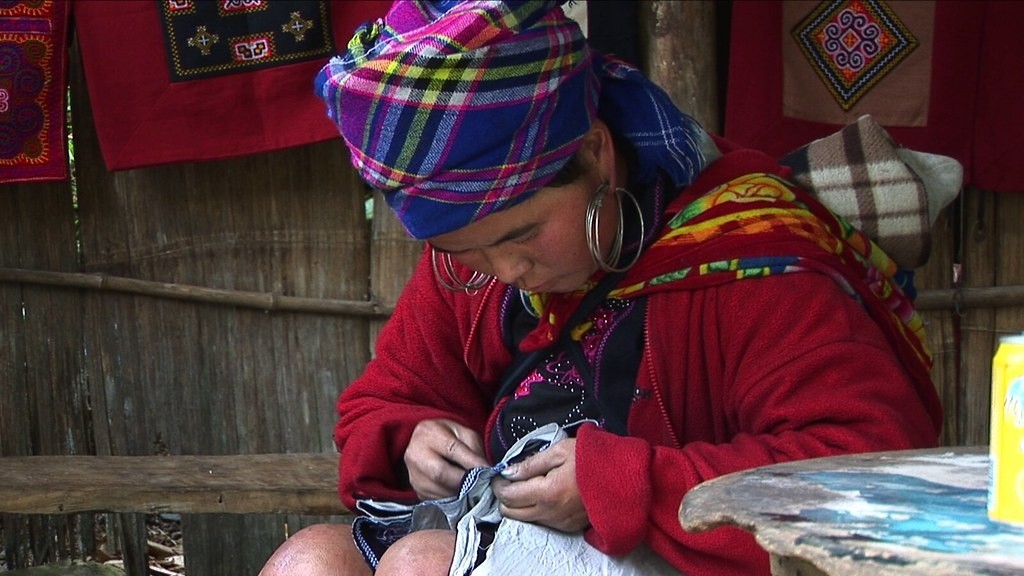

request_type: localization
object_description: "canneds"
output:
[987,334,1024,531]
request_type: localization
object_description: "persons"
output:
[258,0,944,576]
